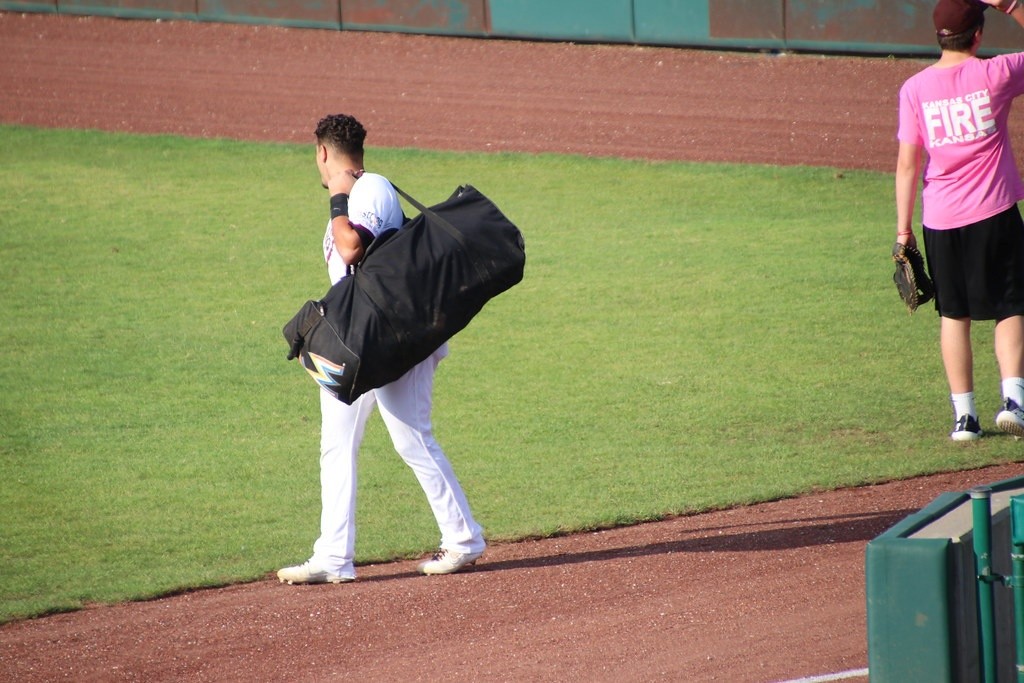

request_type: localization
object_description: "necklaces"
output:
[353,168,365,174]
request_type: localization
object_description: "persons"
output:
[277,113,486,585]
[892,0,1024,444]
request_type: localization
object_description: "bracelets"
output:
[330,193,348,221]
[897,231,911,235]
[1005,0,1018,14]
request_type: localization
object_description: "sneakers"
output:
[277,554,355,587]
[996,396,1024,436]
[414,546,481,576]
[950,413,984,442]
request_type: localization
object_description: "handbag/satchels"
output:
[280,172,525,405]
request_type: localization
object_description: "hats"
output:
[934,0,989,36]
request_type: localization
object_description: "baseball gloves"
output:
[892,242,935,315]
